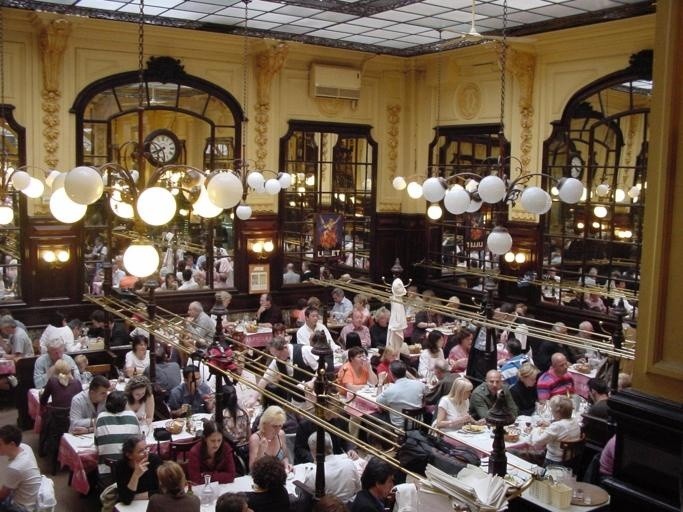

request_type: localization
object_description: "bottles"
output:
[132,367,138,377]
[186,484,195,496]
[200,475,216,512]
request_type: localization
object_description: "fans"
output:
[430,0,546,57]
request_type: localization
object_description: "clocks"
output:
[142,129,183,169]
[565,152,584,184]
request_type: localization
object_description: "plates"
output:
[462,428,486,434]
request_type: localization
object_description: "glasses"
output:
[133,447,150,457]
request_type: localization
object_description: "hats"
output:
[392,277,407,297]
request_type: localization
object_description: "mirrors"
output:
[427,121,510,270]
[66,56,247,296]
[278,117,382,284]
[1,102,30,304]
[532,49,654,290]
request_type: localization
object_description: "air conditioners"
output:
[308,61,362,110]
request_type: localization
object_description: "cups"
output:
[531,464,539,479]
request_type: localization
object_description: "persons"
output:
[0,315,29,334]
[86,231,235,296]
[283,231,370,284]
[0,318,36,430]
[1,423,41,511]
[33,286,631,512]
[0,250,20,300]
[540,262,639,321]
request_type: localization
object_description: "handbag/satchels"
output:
[154,427,171,442]
[467,348,497,380]
[207,340,237,372]
[398,428,481,477]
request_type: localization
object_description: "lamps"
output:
[40,247,70,283]
[550,150,648,239]
[47,0,244,278]
[252,239,275,261]
[233,0,290,224]
[0,1,46,224]
[390,1,584,256]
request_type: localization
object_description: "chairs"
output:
[0,285,636,512]
